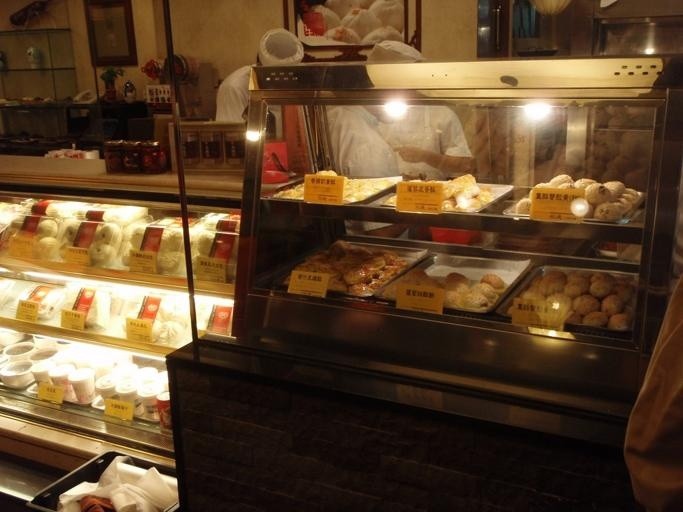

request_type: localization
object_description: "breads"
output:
[1,197,236,286]
[409,171,495,215]
[516,172,642,222]
[274,167,390,205]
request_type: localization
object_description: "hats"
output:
[257,26,305,67]
[370,37,426,64]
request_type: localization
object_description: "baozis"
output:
[296,0,405,47]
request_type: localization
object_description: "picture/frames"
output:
[83,0,138,68]
[283,0,421,63]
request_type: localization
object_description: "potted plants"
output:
[100,69,124,101]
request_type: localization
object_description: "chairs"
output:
[76,99,243,153]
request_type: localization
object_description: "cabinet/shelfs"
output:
[0,28,77,153]
[0,154,244,474]
[231,56,682,407]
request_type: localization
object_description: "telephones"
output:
[72,89,105,105]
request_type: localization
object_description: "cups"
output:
[0,341,61,389]
[29,359,172,432]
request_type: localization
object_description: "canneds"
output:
[181,129,244,167]
[104,139,164,174]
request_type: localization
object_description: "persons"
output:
[317,38,477,181]
[209,29,308,143]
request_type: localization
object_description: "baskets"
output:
[145,83,171,104]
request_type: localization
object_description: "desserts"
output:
[289,238,639,333]
